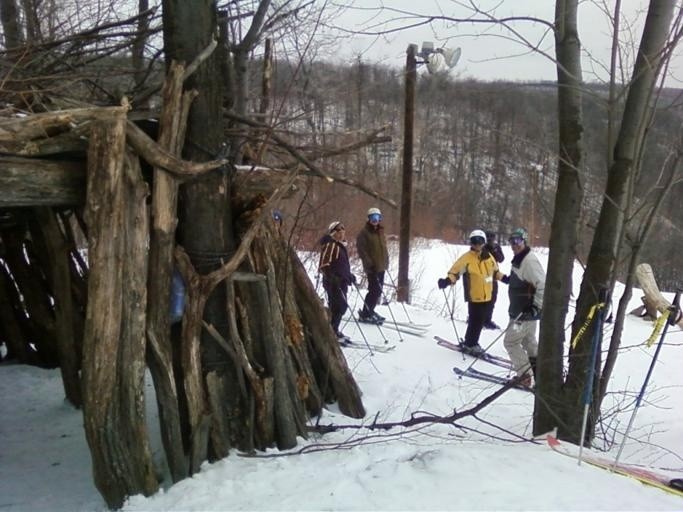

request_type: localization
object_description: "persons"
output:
[447,227,546,386]
[356,208,390,322]
[316,221,355,343]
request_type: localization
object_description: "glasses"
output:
[330,222,345,233]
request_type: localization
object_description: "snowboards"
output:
[548,434,681,497]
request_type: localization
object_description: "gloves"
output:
[439,278,451,289]
[501,275,510,284]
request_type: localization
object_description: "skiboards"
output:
[341,339,397,352]
[342,313,431,334]
[453,367,535,391]
[434,335,516,370]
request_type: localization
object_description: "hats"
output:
[485,229,496,240]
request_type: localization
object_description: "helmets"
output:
[272,210,282,224]
[328,221,345,236]
[508,228,528,245]
[469,229,487,244]
[367,207,383,222]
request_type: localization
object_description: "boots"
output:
[358,309,386,325]
[509,374,531,387]
[459,343,481,355]
[335,332,353,346]
